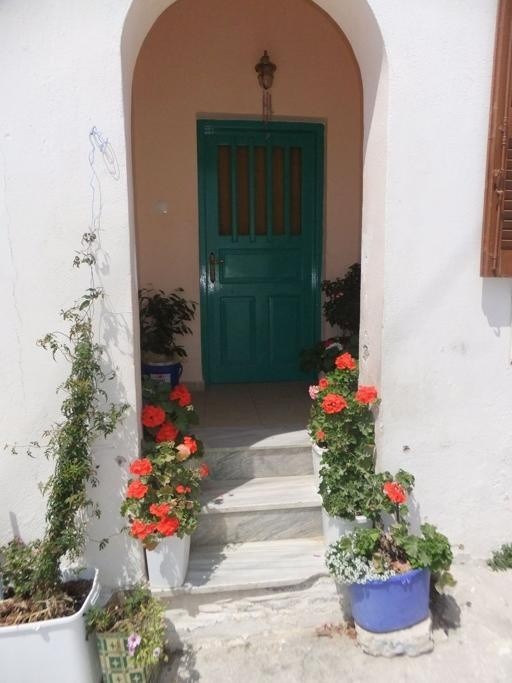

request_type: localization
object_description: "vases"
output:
[345,568,430,633]
[141,532,191,589]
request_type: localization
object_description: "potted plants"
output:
[136,283,198,390]
[85,573,167,683]
[3,234,108,683]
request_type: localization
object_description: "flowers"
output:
[308,354,456,594]
[122,381,207,548]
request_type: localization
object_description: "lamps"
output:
[255,49,276,122]
